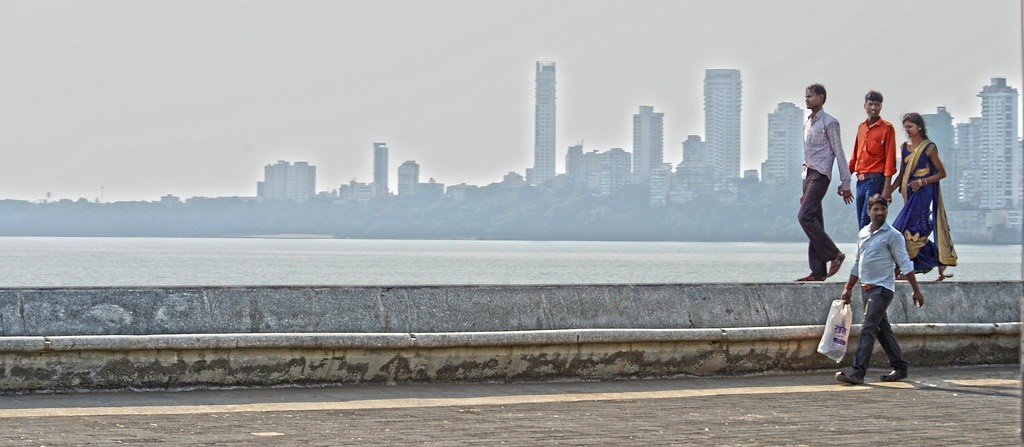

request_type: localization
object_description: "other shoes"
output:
[835,368,864,384]
[797,273,827,281]
[880,369,907,381]
[827,251,845,277]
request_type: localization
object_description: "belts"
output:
[860,283,874,290]
[856,173,881,180]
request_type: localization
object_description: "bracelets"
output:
[916,179,928,186]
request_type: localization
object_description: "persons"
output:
[835,193,924,384]
[891,112,958,281]
[837,91,898,231]
[797,82,854,282]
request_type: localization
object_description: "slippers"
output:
[936,274,953,281]
[896,274,909,279]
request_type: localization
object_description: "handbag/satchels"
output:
[817,300,852,364]
